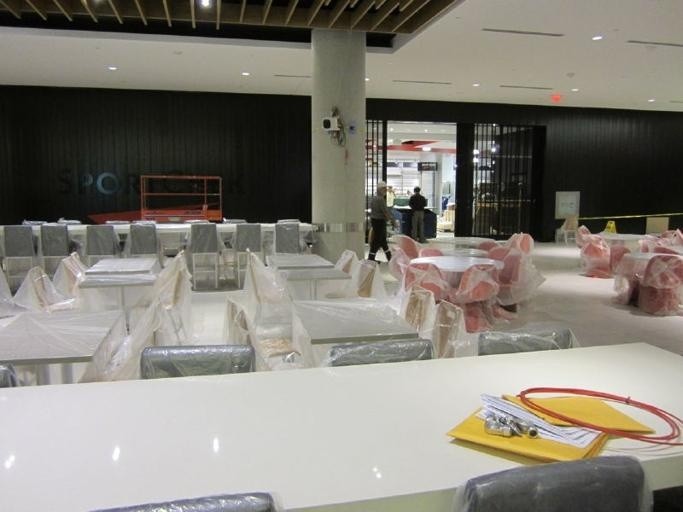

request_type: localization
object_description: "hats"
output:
[377,181,390,188]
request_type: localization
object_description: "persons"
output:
[367,180,392,264]
[407,187,428,245]
[387,186,395,212]
